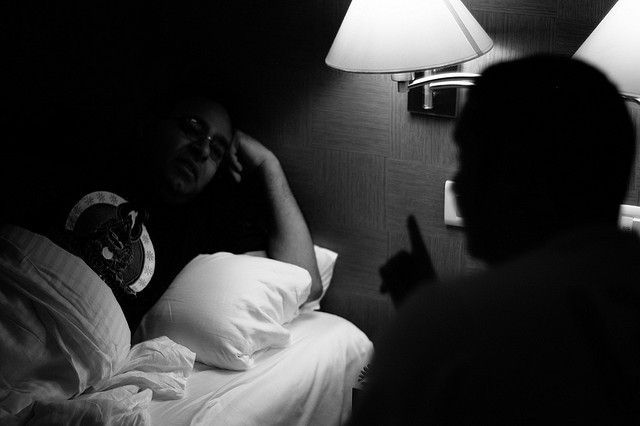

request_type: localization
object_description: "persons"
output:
[353,52,637,426]
[48,102,323,333]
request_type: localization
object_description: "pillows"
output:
[135,251,312,372]
[240,244,338,312]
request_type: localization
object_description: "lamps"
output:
[570,0,640,107]
[324,0,494,112]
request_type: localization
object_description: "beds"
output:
[145,312,376,426]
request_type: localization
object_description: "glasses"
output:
[177,116,230,164]
